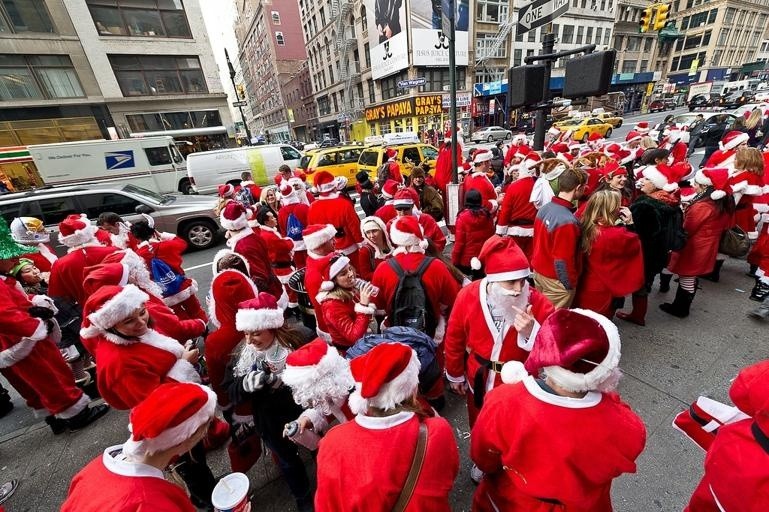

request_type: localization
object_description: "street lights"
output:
[224,50,252,146]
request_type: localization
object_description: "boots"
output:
[699,259,725,283]
[615,296,648,326]
[745,264,769,302]
[659,272,699,317]
[173,460,209,508]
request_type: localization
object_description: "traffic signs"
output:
[398,78,425,87]
[233,101,246,107]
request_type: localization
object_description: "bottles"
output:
[36,272,49,279]
[283,421,321,451]
[351,278,380,297]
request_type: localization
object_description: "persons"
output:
[431,0,468,30]
[680,362,769,512]
[469,308,646,512]
[374,0,401,44]
[80,284,215,512]
[315,342,459,512]
[60,382,251,512]
[223,293,357,512]
[1,95,768,432]
[2,479,18,503]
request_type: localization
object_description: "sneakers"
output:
[469,463,485,485]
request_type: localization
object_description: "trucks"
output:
[26,135,197,196]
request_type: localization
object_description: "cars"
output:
[358,144,466,196]
[298,145,384,195]
[472,126,512,144]
[649,78,769,147]
[256,128,419,149]
[523,110,623,144]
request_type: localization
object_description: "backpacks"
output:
[385,256,436,337]
[150,244,186,296]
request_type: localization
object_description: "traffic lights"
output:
[639,9,650,32]
[658,5,670,27]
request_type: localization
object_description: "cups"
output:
[265,346,288,375]
[211,472,250,512]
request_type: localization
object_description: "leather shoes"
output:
[45,415,68,435]
[66,403,109,433]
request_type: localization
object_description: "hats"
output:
[218,165,428,410]
[508,122,696,193]
[384,147,398,160]
[443,125,463,143]
[0,217,40,260]
[79,249,150,339]
[470,234,530,283]
[471,149,494,165]
[717,129,751,152]
[121,382,216,462]
[695,167,729,201]
[500,306,621,394]
[59,212,94,248]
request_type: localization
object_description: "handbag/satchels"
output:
[671,395,753,454]
[229,425,262,473]
[718,225,750,258]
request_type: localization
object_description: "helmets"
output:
[10,217,50,244]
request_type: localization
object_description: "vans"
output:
[185,144,303,196]
[0,183,225,250]
